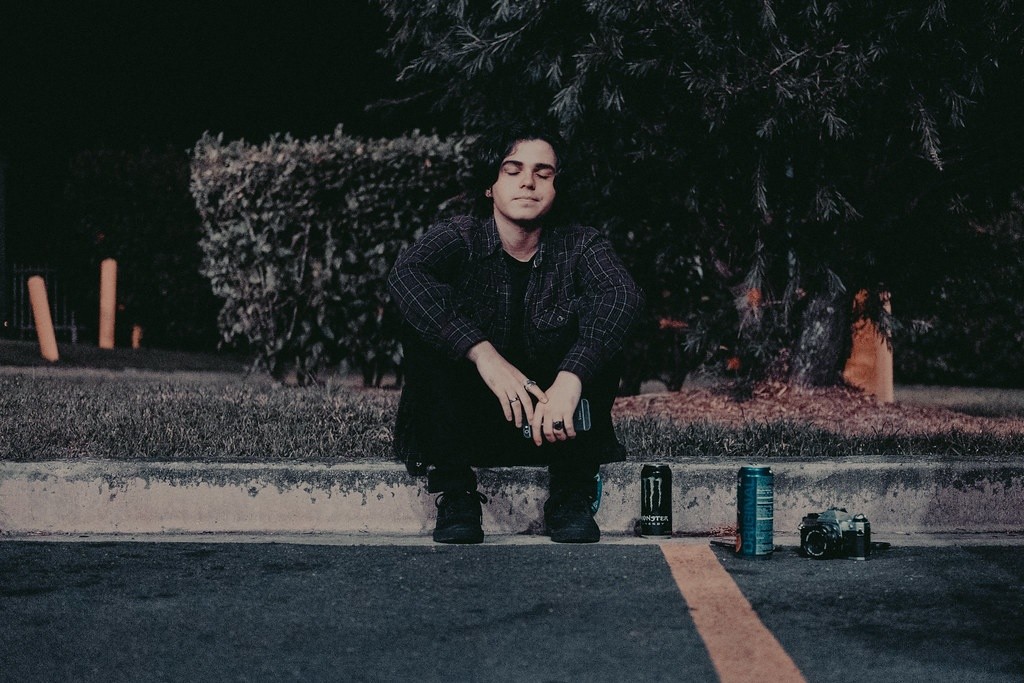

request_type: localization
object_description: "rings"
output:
[553,421,564,430]
[524,379,536,391]
[509,396,519,404]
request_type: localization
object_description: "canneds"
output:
[734,467,775,559]
[640,463,672,539]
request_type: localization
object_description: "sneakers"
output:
[434,477,487,544]
[544,475,601,543]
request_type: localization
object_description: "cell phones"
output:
[522,397,590,439]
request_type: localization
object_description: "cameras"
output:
[796,506,870,560]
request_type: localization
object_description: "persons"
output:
[388,118,642,544]
